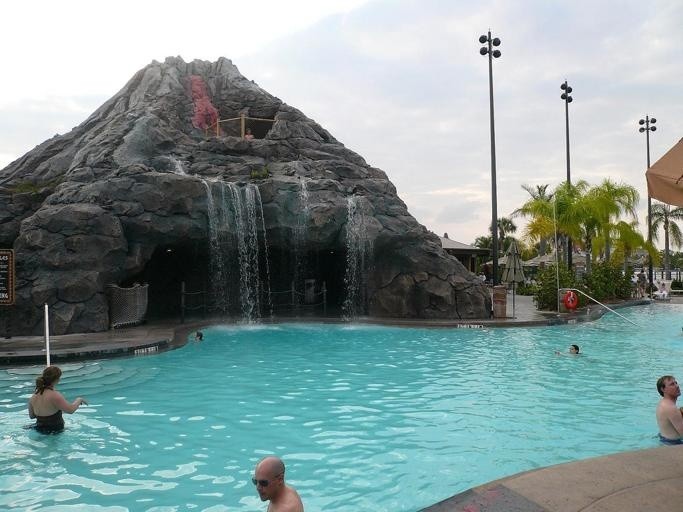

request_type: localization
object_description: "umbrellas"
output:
[501,239,526,317]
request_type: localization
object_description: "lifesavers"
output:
[564,291,578,309]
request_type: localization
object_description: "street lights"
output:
[478,28,503,286]
[559,80,574,274]
[638,115,657,284]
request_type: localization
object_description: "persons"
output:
[555,344,579,357]
[654,375,682,445]
[194,332,203,342]
[636,269,647,297]
[28,366,88,435]
[660,283,669,299]
[245,128,254,139]
[252,455,304,512]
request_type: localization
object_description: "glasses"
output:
[251,472,282,487]
